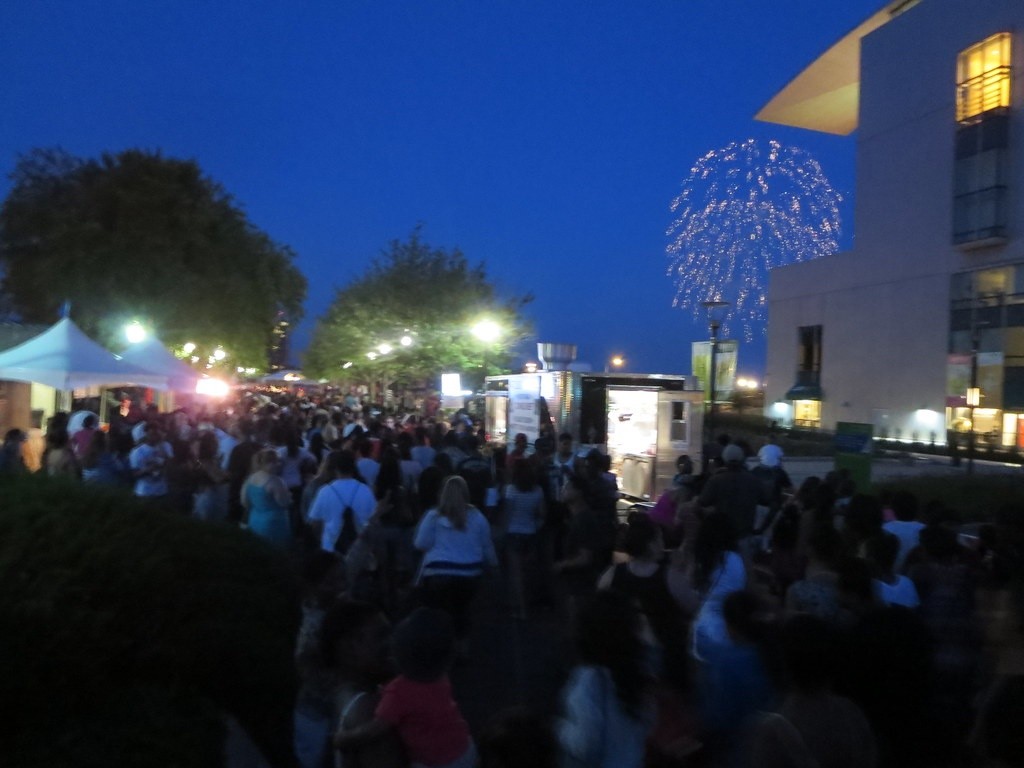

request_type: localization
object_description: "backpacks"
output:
[325,481,362,556]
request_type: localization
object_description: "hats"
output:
[513,433,528,441]
[392,604,462,685]
[721,444,743,464]
[757,444,784,459]
[535,438,549,449]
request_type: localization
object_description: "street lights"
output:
[699,301,729,445]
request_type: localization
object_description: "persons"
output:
[0,389,1024,768]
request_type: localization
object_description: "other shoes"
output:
[512,611,527,620]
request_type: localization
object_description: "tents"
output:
[1,319,168,422]
[112,338,228,397]
